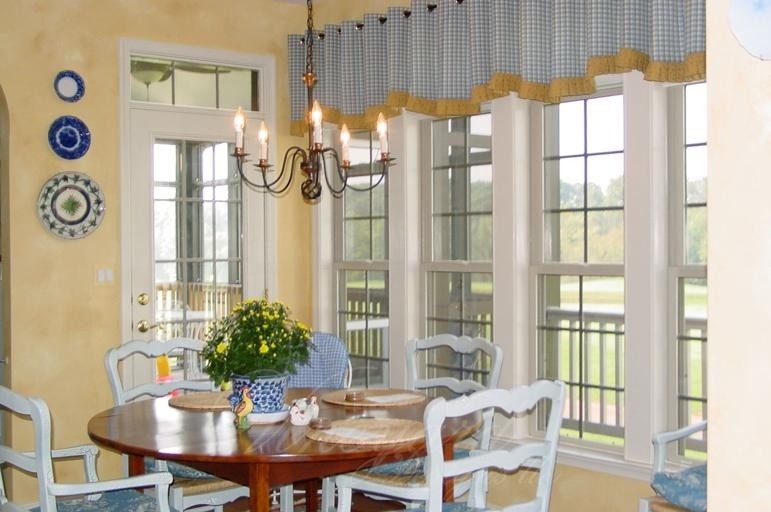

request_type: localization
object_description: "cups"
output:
[346,391,365,402]
[309,417,332,429]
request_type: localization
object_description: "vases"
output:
[229,371,292,412]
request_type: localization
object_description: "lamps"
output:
[212,0,397,203]
[129,60,171,88]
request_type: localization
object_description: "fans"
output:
[159,61,232,85]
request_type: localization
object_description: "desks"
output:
[155,307,213,326]
[84,386,488,511]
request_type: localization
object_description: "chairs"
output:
[632,413,707,512]
[280,326,357,510]
[331,374,569,511]
[156,297,196,360]
[1,383,179,511]
[100,337,295,510]
[324,335,504,511]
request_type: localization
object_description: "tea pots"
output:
[290,395,321,426]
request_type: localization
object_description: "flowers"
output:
[197,296,322,393]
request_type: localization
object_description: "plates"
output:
[232,402,290,426]
[36,171,106,241]
[48,116,92,161]
[51,69,86,103]
